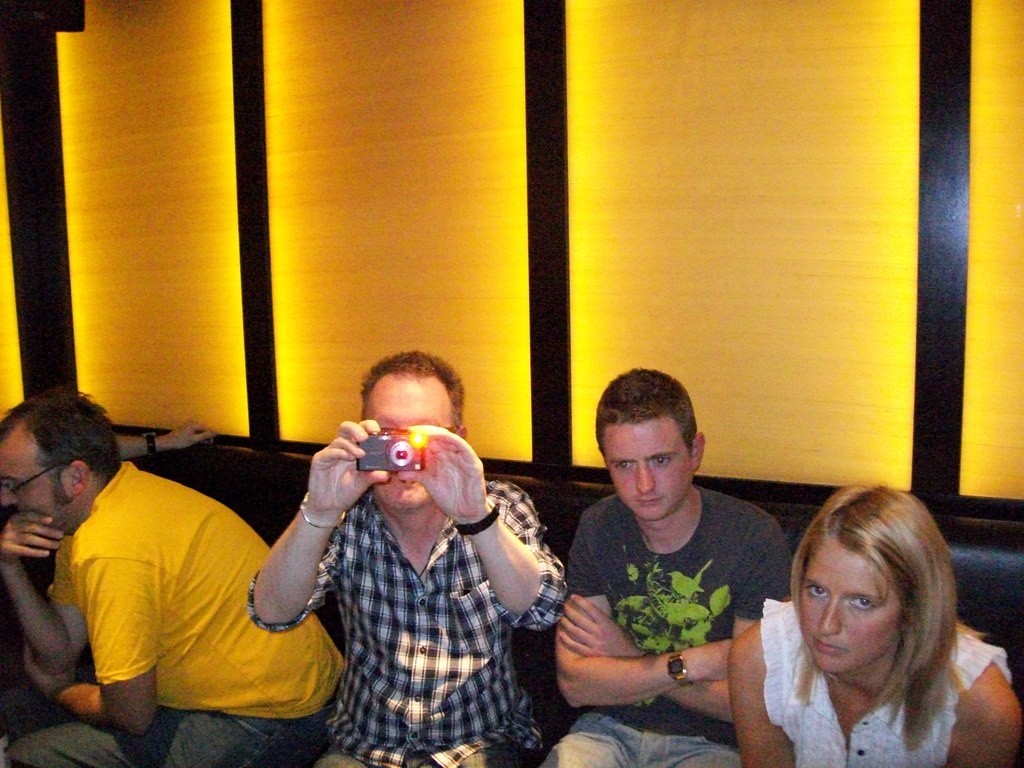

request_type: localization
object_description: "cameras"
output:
[356,427,426,471]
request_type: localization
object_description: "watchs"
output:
[143,431,157,457]
[667,650,694,688]
[453,497,501,536]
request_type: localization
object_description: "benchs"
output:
[203,440,1024,768]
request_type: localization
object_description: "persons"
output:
[0,387,344,768]
[245,351,567,768]
[726,484,1023,768]
[0,420,217,746]
[538,369,793,768]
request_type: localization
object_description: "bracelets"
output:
[300,492,346,529]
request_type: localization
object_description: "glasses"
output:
[0,461,72,499]
